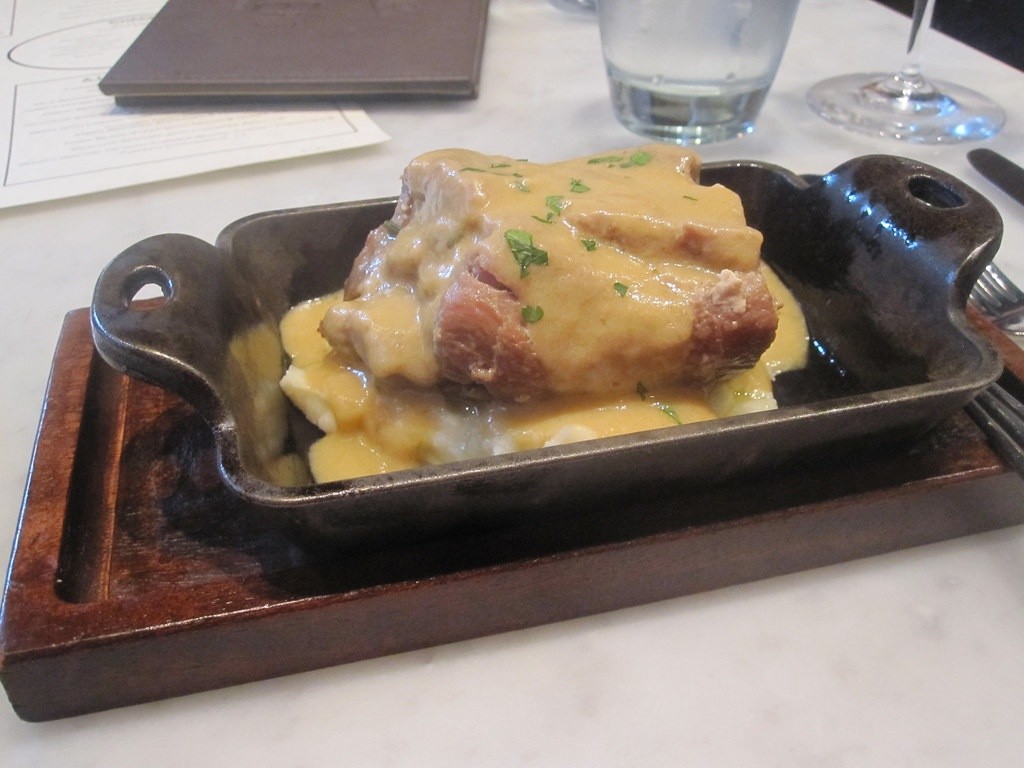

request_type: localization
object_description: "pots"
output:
[86,151,1006,539]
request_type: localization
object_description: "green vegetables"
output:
[459,149,699,323]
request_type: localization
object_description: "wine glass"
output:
[805,0,1007,146]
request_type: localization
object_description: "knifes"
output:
[966,148,1024,207]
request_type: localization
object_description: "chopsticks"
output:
[963,390,1024,478]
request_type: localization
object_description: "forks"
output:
[969,260,1024,336]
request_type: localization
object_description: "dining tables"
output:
[3,0,1024,767]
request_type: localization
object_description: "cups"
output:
[594,1,802,146]
